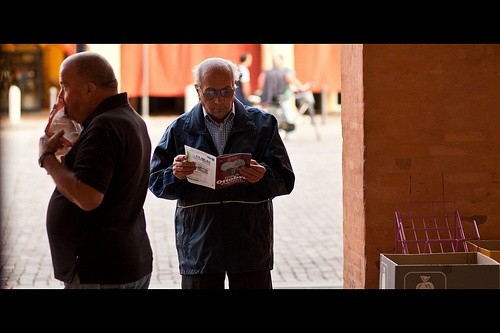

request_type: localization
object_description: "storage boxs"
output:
[468,239,500,262]
[380,251,500,289]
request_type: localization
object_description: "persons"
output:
[255,53,306,125]
[235,53,254,107]
[148,58,295,289]
[37,51,153,289]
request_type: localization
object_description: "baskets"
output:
[298,91,315,106]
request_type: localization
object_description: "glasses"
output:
[200,86,235,99]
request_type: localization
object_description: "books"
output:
[184,145,252,190]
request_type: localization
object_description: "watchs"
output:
[38,151,56,168]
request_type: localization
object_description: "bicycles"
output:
[250,83,321,143]
